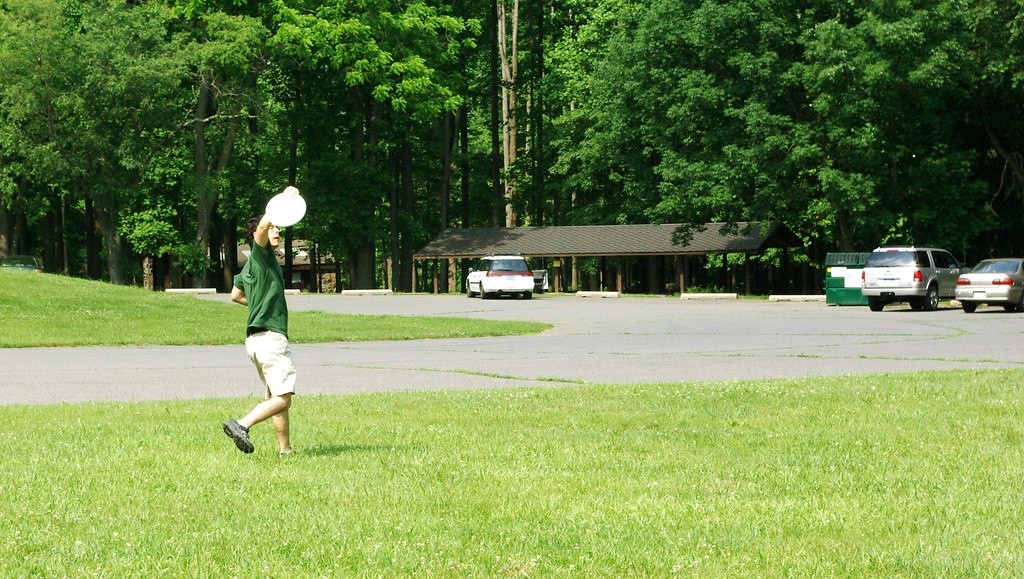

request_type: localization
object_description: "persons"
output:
[221,186,300,455]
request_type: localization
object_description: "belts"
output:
[251,328,269,335]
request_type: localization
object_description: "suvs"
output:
[861,245,965,313]
[465,253,536,299]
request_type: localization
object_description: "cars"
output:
[954,258,1024,315]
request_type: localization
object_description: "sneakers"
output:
[222,420,254,454]
[279,447,298,458]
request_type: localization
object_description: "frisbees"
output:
[265,192,306,227]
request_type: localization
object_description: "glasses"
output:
[269,224,281,232]
